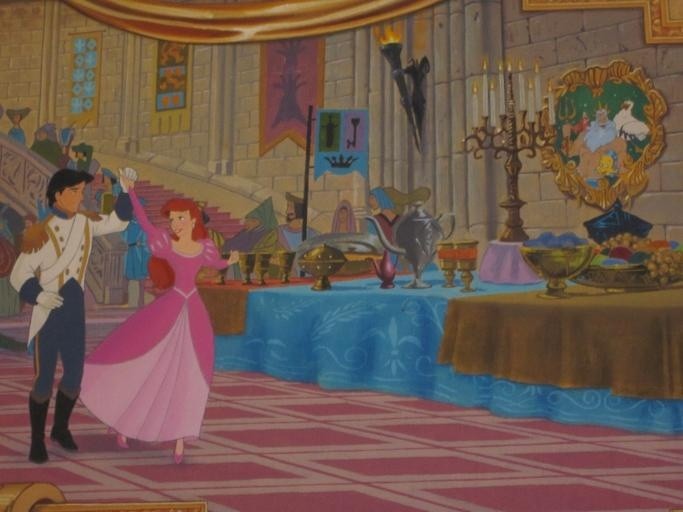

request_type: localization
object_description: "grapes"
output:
[594,233,683,286]
